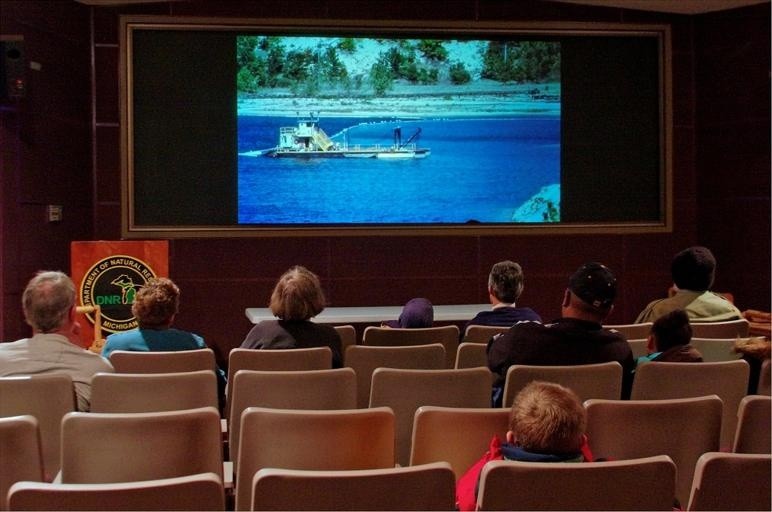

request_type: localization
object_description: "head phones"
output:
[634,351,661,367]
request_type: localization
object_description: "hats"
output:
[670,245,717,292]
[567,261,618,310]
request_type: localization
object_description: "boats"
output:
[266,107,433,161]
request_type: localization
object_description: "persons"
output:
[100,278,228,411]
[453,379,685,511]
[460,260,545,339]
[239,264,345,369]
[380,299,435,328]
[636,245,742,322]
[0,271,115,411]
[487,262,633,407]
[632,308,708,377]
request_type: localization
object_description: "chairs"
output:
[59,406,234,488]
[731,394,771,454]
[1,414,62,486]
[5,472,226,511]
[582,394,726,511]
[409,405,512,480]
[2,316,771,454]
[475,453,678,511]
[686,452,771,512]
[250,461,457,511]
[234,406,402,511]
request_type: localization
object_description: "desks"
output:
[244,303,496,327]
